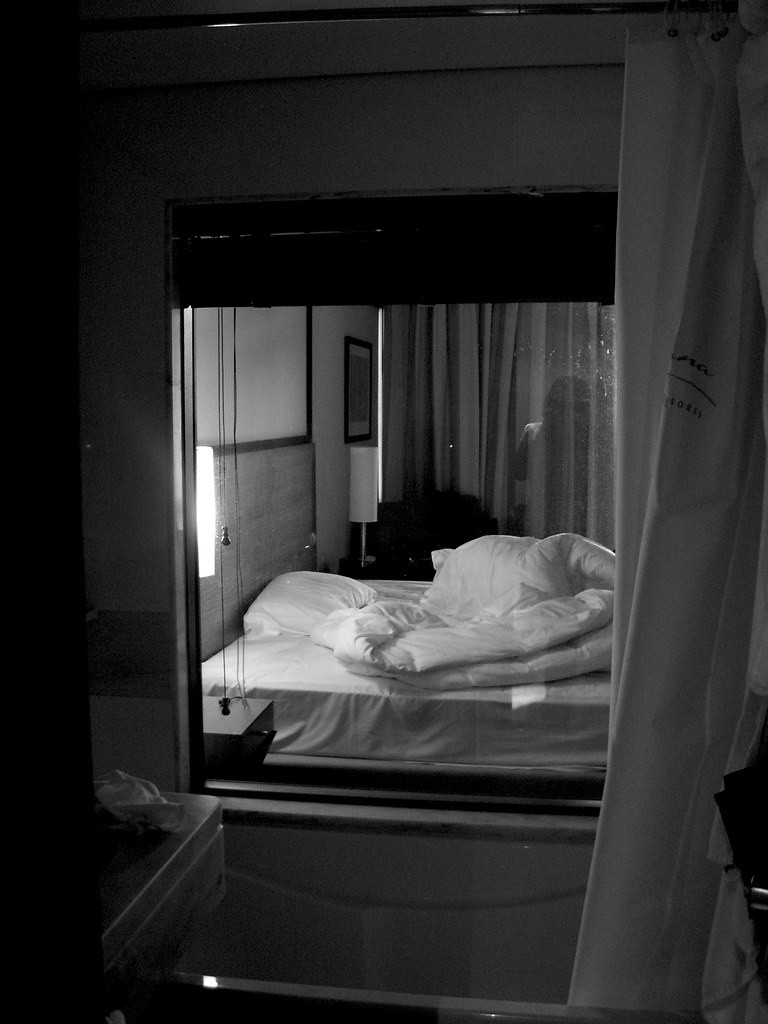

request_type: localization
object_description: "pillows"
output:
[243,570,379,637]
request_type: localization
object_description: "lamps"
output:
[351,447,380,568]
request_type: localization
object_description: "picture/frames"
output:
[345,336,373,444]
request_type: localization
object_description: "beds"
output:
[203,532,617,771]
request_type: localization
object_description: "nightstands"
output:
[204,697,277,782]
[96,792,228,979]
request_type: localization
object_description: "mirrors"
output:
[164,188,617,814]
